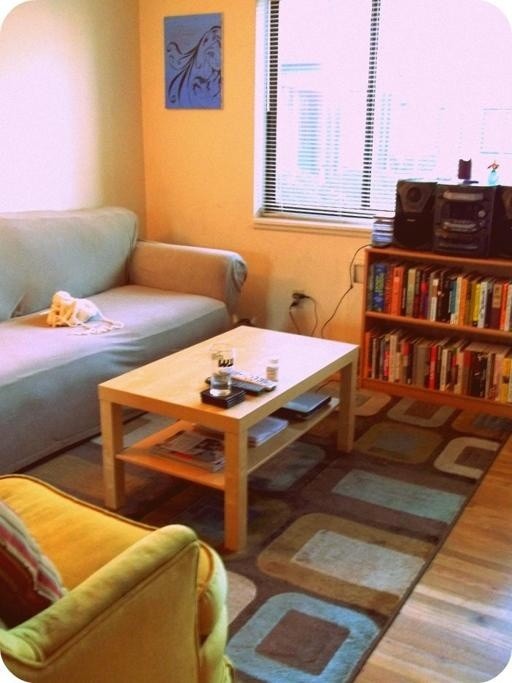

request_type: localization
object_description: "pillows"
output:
[0,503,63,626]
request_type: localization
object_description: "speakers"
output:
[492,185,511,260]
[391,177,440,252]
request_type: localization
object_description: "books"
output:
[362,256,512,405]
[153,414,288,472]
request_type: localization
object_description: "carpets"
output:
[13,378,509,682]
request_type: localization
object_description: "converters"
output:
[292,293,305,299]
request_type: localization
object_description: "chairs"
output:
[0,469,235,681]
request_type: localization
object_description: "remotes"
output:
[205,375,263,395]
[219,367,277,392]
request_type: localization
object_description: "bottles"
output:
[266,357,280,385]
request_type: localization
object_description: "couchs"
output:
[0,207,248,474]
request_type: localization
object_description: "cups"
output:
[209,366,234,397]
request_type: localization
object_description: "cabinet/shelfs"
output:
[356,245,510,418]
